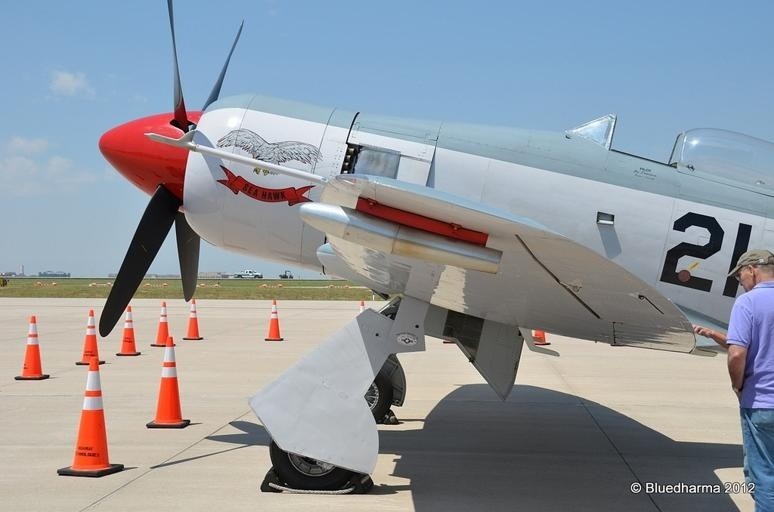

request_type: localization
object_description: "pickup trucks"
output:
[232,269,264,278]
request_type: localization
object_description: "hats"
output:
[728,249,774,276]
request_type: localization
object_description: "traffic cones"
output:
[114,306,142,357]
[13,313,52,380]
[179,298,206,341]
[147,299,176,349]
[144,333,191,430]
[533,327,552,346]
[357,299,366,314]
[55,354,128,478]
[263,296,286,341]
[73,306,107,365]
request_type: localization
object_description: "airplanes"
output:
[93,0,774,501]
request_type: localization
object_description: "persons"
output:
[692,248,774,512]
